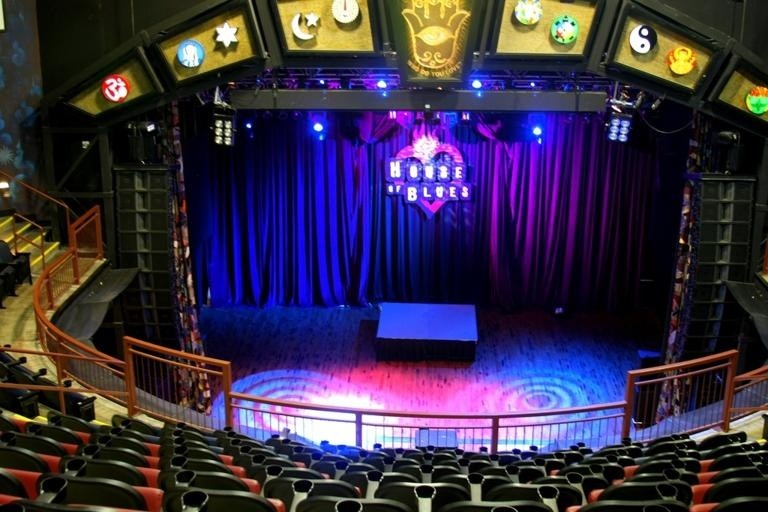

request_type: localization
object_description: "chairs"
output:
[1,239,34,310]
[1,343,767,510]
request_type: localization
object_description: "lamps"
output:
[214,113,236,146]
[601,98,638,145]
[525,111,550,146]
[471,75,489,92]
[306,112,328,142]
[244,120,254,130]
[373,75,390,90]
[143,120,156,133]
